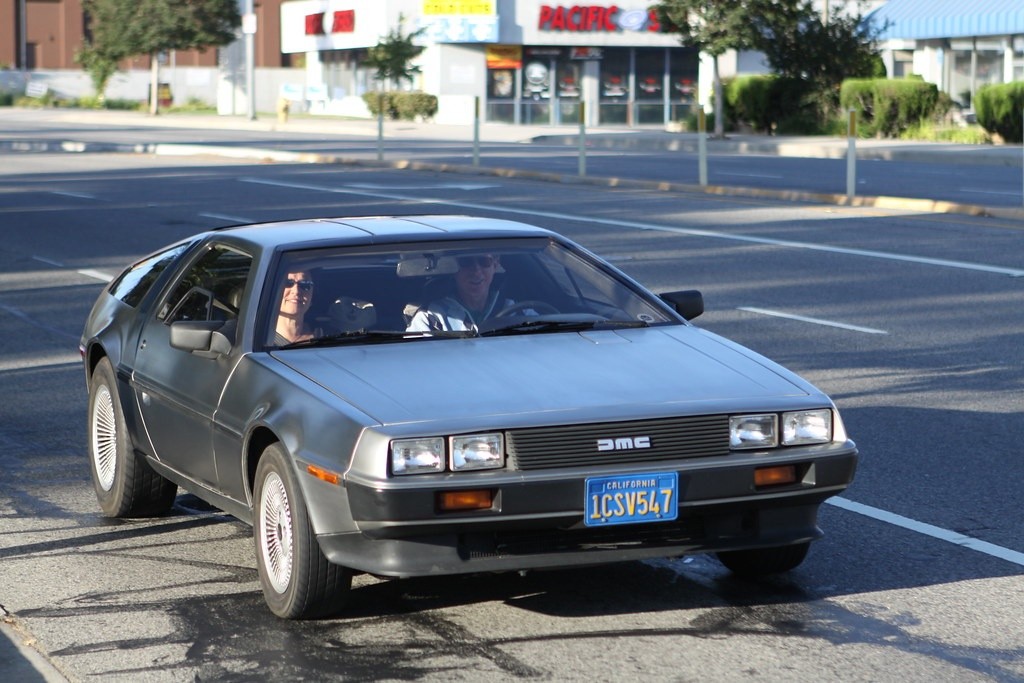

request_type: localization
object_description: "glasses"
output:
[285,280,314,291]
[458,256,492,267]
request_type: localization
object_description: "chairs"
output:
[224,267,455,335]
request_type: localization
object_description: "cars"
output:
[79,212,860,621]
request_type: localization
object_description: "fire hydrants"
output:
[273,96,293,126]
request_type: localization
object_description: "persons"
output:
[402,249,539,338]
[272,262,334,346]
